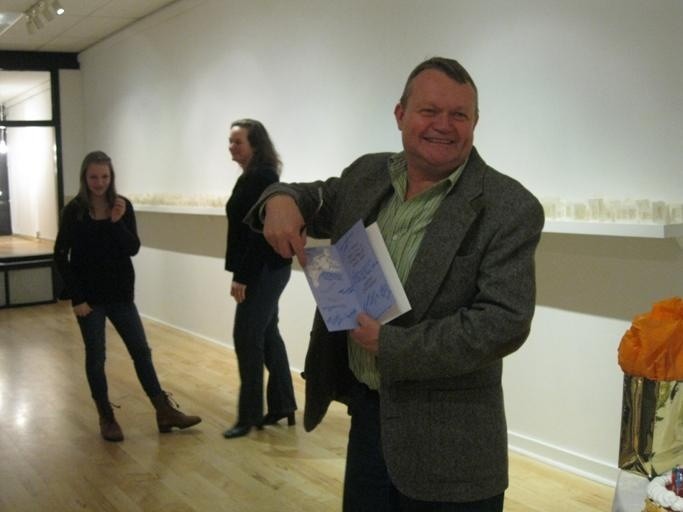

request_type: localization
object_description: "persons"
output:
[233,56,545,512]
[50,150,203,442]
[215,118,295,440]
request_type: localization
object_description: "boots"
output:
[149,389,202,434]
[91,395,125,442]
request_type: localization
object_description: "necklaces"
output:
[93,204,109,211]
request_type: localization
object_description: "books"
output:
[289,216,413,336]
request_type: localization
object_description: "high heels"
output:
[222,411,266,439]
[261,408,297,427]
